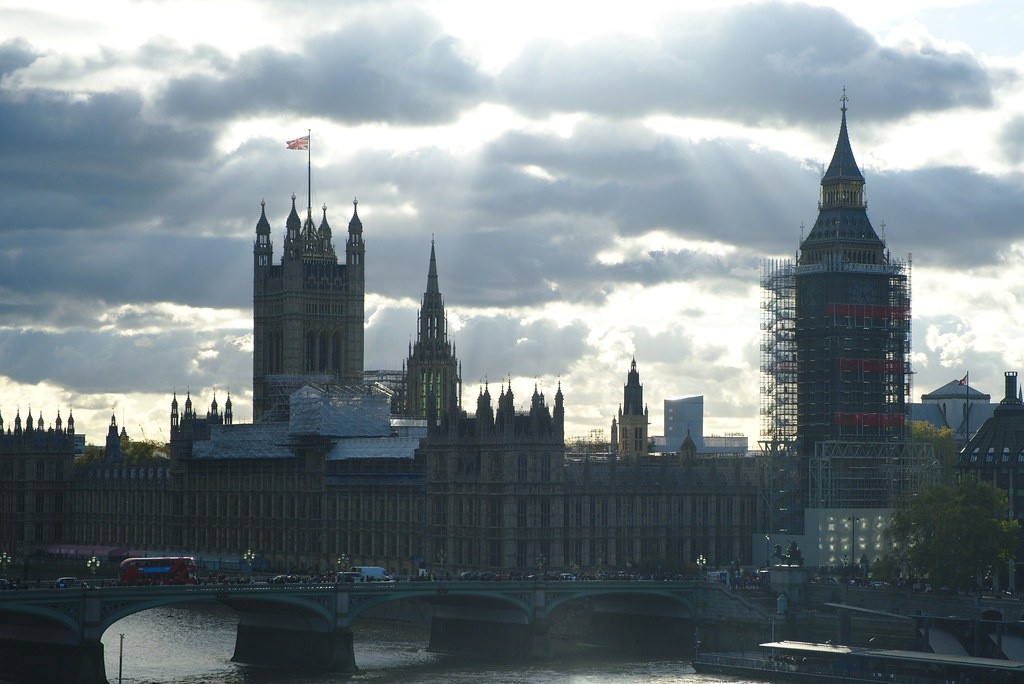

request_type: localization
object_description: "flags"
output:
[287,136,308,150]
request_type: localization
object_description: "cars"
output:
[54,577,89,588]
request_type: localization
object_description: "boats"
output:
[693,637,1024,684]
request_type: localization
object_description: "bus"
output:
[118,556,197,586]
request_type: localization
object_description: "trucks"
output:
[337,567,390,581]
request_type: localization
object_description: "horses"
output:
[771,544,788,566]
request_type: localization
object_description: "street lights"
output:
[0,552,11,579]
[848,515,860,576]
[765,533,770,567]
[87,557,100,577]
[338,553,349,581]
[696,554,706,575]
[243,549,255,576]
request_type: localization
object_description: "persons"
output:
[408,570,534,581]
[731,574,760,589]
[548,569,711,582]
[193,575,255,585]
[0,573,184,590]
[266,574,369,585]
